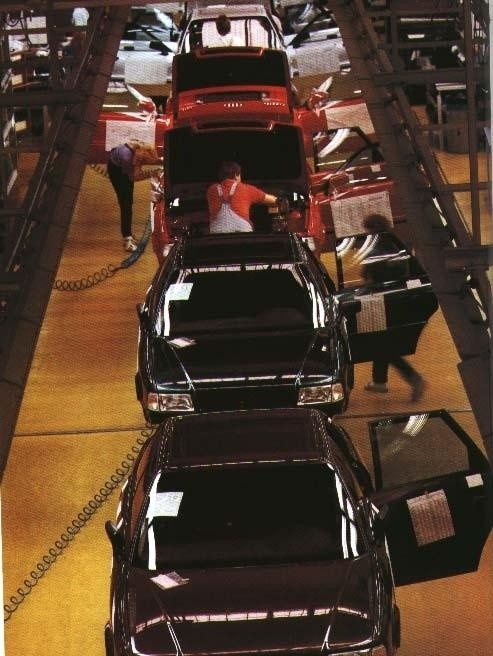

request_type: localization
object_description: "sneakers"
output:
[411,380,428,402]
[124,236,141,251]
[364,381,389,392]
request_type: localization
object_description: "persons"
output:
[105,136,164,253]
[360,212,437,405]
[202,159,291,235]
